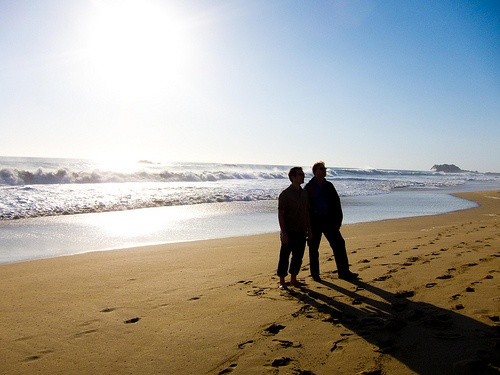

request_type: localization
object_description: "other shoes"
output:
[279,283,287,289]
[338,271,358,279]
[313,274,321,281]
[290,280,302,286]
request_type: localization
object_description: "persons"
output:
[303,160,359,281]
[278,162,312,289]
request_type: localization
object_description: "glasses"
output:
[318,168,327,170]
[295,173,305,176]
